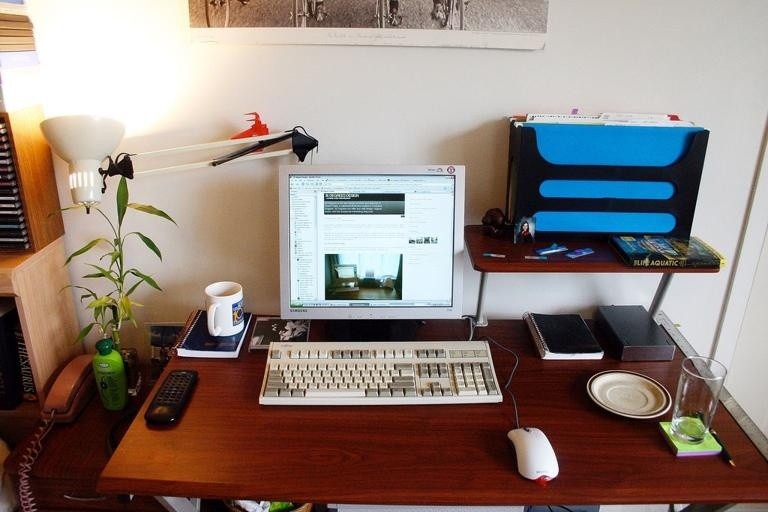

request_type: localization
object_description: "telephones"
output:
[40,354,98,423]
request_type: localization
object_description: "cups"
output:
[204,280,246,337]
[670,355,728,444]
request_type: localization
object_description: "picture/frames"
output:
[179,0,552,52]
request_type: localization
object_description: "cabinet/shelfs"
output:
[0,237,165,512]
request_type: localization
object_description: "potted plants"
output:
[47,175,178,396]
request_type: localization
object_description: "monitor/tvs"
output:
[278,165,465,342]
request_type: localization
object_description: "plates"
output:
[586,368,673,419]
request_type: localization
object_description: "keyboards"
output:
[258,342,504,406]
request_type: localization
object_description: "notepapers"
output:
[660,418,723,456]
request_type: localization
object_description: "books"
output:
[171,308,253,360]
[521,310,604,362]
[607,234,728,267]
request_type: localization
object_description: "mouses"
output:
[507,427,559,481]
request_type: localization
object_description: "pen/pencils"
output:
[483,253,505,258]
[708,429,736,467]
[525,255,548,260]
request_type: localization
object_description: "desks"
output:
[98,308,768,512]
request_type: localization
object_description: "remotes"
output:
[144,368,198,425]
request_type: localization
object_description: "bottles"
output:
[91,340,127,410]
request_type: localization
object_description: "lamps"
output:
[40,111,319,215]
